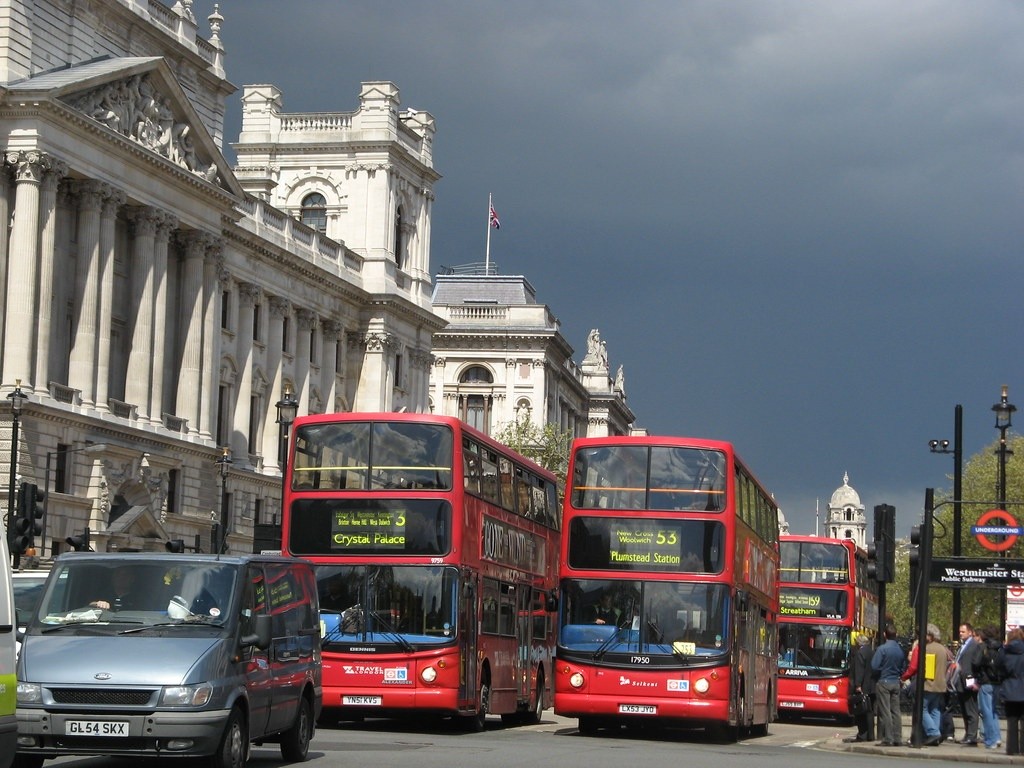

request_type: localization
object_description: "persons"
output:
[90,565,158,611]
[972,627,1001,750]
[71,76,222,185]
[955,623,984,743]
[583,593,617,626]
[614,364,624,390]
[994,629,1024,755]
[871,625,906,746]
[903,624,957,747]
[848,633,878,742]
[517,403,529,426]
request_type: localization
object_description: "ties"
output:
[955,643,965,661]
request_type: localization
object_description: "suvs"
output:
[15,551,323,768]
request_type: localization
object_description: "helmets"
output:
[167,595,194,620]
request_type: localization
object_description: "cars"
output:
[11,568,68,666]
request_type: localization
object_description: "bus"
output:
[282,412,562,734]
[552,436,781,744]
[777,535,884,726]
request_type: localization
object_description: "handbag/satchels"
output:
[847,690,872,716]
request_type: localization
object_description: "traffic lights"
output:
[7,515,31,555]
[909,523,921,568]
[867,540,886,583]
[23,483,45,537]
[65,534,85,553]
[213,524,230,554]
[166,539,185,553]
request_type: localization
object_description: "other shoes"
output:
[908,733,1005,751]
[875,741,889,746]
[850,735,873,742]
[892,740,903,747]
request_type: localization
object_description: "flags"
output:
[490,204,500,230]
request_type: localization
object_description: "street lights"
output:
[275,387,300,553]
[989,385,1019,646]
[5,377,30,564]
[213,447,234,555]
